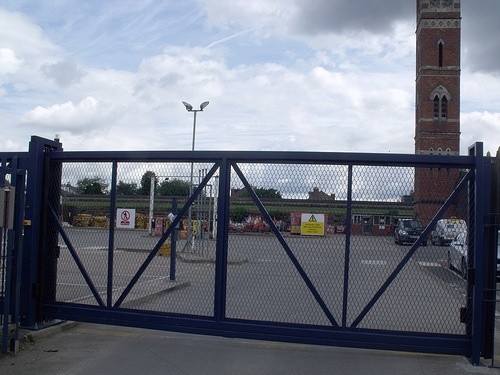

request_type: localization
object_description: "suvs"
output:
[432,215,467,245]
[394,218,427,244]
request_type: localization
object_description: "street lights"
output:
[180,100,209,254]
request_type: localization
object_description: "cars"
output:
[447,228,500,281]
